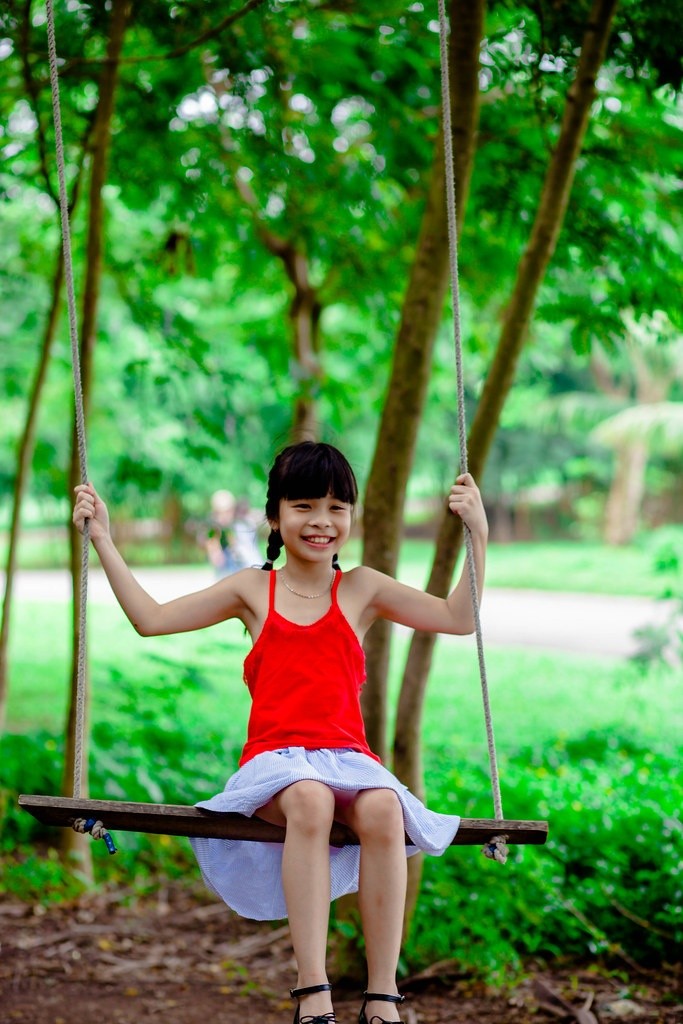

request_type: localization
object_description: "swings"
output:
[10,1,551,868]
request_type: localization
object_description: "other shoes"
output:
[289,983,339,1024]
[358,992,406,1024]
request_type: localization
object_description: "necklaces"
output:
[282,568,335,599]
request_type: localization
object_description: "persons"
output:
[73,441,488,1024]
